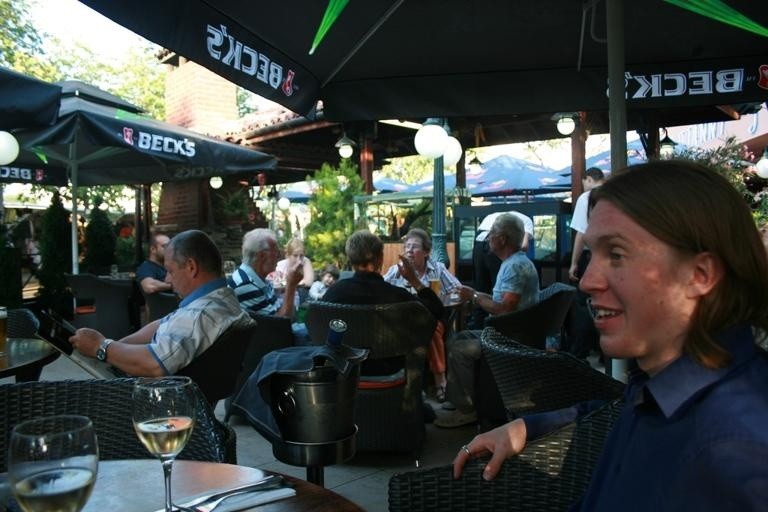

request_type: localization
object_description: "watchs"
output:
[434,215,540,428]
[96,338,114,360]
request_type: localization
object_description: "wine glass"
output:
[6,414,99,512]
[223,260,236,280]
[131,375,200,512]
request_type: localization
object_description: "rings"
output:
[462,443,471,456]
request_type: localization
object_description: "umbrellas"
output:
[1,67,62,131]
[0,97,278,310]
[80,0,768,388]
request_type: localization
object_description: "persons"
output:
[70,228,255,377]
[315,230,445,321]
[134,228,339,323]
[568,167,606,358]
[472,210,537,329]
[453,159,768,511]
[382,228,462,402]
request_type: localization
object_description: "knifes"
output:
[154,475,285,512]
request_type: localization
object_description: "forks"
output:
[170,482,298,512]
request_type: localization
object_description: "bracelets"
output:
[471,293,478,305]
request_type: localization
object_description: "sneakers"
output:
[434,410,479,427]
[443,402,456,408]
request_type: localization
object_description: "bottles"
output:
[316,318,347,366]
[294,290,304,324]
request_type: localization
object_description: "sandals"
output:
[435,384,446,402]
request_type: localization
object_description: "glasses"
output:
[405,243,423,248]
[263,247,281,259]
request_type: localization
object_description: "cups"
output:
[110,264,118,278]
[0,306,10,357]
[428,279,441,297]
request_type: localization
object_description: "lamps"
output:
[660,127,678,146]
[0,129,20,167]
[440,117,463,166]
[268,187,278,201]
[551,112,581,136]
[334,129,356,158]
[413,117,448,161]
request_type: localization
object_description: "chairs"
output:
[1,376,237,466]
[304,301,439,461]
[6,309,41,339]
[467,282,579,350]
[387,399,637,510]
[140,288,178,323]
[67,273,103,330]
[93,279,139,342]
[223,308,292,425]
[178,312,256,410]
[480,325,629,422]
